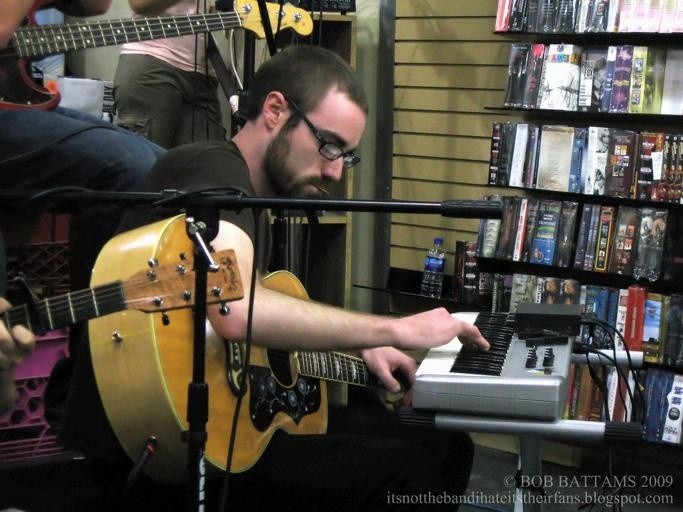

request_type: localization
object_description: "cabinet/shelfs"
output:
[475,0,682,511]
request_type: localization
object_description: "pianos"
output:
[415,310,574,422]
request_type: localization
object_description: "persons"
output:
[59,45,490,512]
[0,1,167,294]
[113,1,224,149]
[0,297,36,414]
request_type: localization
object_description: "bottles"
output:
[420,238,446,298]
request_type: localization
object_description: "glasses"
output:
[283,95,360,168]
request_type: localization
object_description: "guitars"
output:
[0,251,242,344]
[0,0,314,65]
[86,213,423,475]
